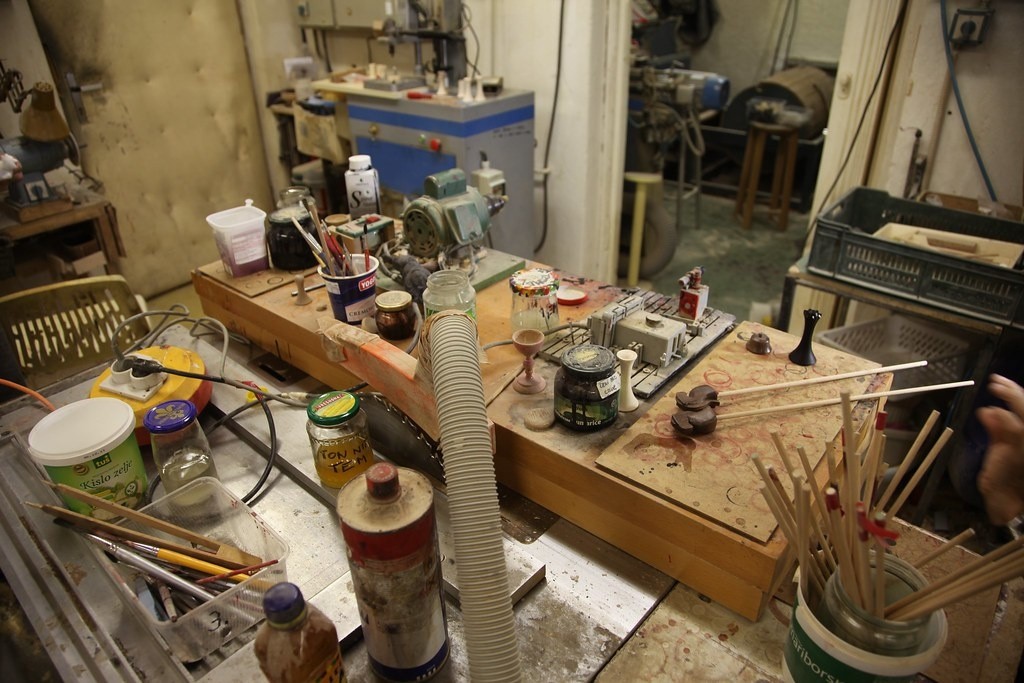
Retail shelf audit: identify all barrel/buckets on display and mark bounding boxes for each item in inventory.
[26,396,149,523]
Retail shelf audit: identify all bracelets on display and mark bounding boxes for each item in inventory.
[992,522,1024,545]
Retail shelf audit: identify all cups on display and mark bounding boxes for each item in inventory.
[318,253,382,325]
[780,545,947,683]
[509,266,559,341]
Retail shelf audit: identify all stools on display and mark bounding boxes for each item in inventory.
[734,119,798,232]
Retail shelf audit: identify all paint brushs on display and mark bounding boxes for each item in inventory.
[289,196,359,277]
[51,518,256,626]
[363,224,369,271]
[117,538,274,590]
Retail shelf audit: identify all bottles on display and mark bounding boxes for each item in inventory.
[818,557,933,657]
[305,391,374,491]
[422,270,477,325]
[252,581,348,683]
[344,154,382,220]
[142,400,220,507]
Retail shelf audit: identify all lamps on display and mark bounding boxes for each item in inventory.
[0,59,70,141]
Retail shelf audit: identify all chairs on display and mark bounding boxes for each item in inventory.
[0,274,153,389]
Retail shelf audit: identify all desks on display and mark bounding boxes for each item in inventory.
[0,186,123,282]
[778,248,1005,526]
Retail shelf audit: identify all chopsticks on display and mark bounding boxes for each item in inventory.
[748,386,1024,629]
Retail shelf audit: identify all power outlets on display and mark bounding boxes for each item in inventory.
[948,7,992,46]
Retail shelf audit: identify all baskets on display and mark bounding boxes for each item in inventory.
[808,187,1024,321]
[816,314,972,400]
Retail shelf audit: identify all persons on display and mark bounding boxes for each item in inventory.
[978,374,1024,548]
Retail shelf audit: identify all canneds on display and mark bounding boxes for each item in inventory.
[307,390,374,489]
[553,344,620,431]
[374,291,415,340]
[265,207,323,270]
[141,400,220,508]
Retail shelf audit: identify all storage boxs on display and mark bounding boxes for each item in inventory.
[89,477,291,664]
[815,184,1024,321]
[817,312,978,401]
[204,203,269,275]
